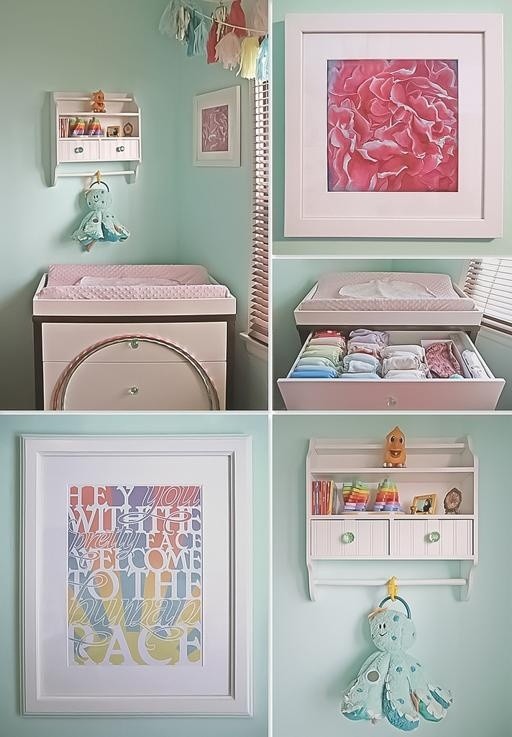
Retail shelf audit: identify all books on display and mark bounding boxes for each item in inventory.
[313,479,338,514]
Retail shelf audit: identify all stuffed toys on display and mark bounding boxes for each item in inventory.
[72,188,130,250]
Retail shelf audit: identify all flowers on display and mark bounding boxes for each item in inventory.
[328,60,460,192]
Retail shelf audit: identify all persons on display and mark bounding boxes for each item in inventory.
[340,609,452,731]
[424,499,431,510]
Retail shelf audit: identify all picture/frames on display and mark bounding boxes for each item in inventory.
[284,9,506,240]
[408,490,438,516]
[17,433,255,718]
[191,84,243,169]
[106,125,120,137]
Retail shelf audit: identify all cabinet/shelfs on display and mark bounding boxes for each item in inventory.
[300,429,483,608]
[30,264,237,408]
[41,88,142,188]
[275,266,509,410]
